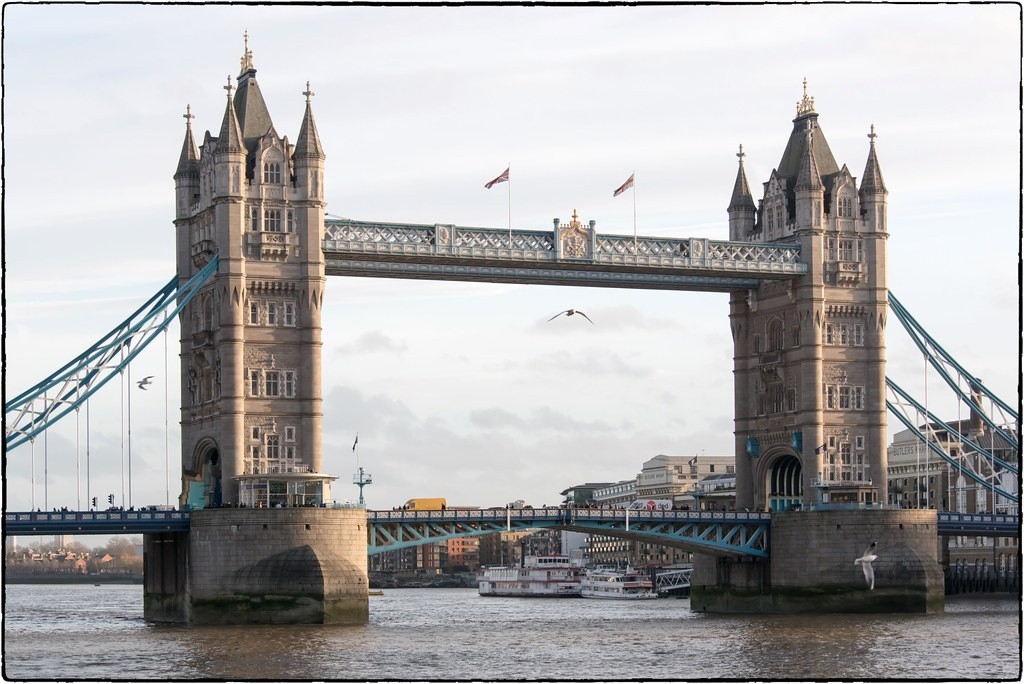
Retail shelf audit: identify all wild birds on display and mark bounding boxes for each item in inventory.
[853,541,879,590]
[136,375,155,391]
[546,308,596,326]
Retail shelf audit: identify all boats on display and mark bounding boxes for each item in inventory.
[580,564,658,599]
[476,554,584,597]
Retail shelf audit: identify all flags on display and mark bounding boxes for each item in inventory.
[689,457,697,466]
[352,436,358,451]
[613,175,634,197]
[485,168,509,189]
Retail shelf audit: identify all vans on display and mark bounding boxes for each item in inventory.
[403,498,447,511]
[625,499,672,511]
